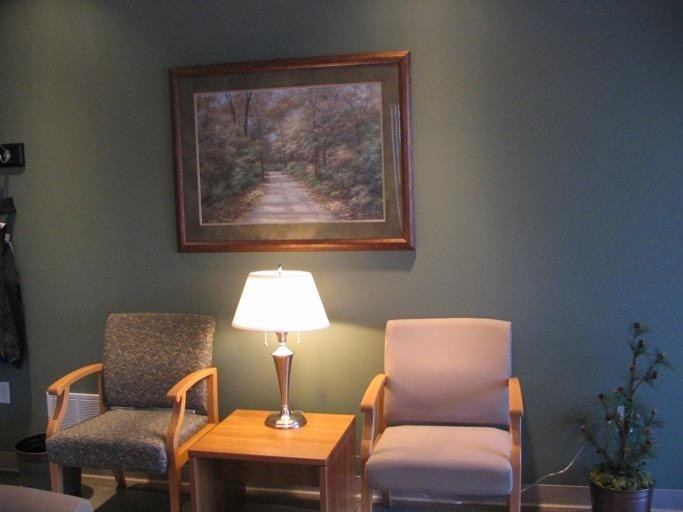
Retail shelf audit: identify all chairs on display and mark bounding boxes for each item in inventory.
[359,317,526,512]
[44,313,220,512]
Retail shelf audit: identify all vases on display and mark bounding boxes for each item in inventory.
[589,477,654,512]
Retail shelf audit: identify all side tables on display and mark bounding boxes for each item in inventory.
[186,408,359,512]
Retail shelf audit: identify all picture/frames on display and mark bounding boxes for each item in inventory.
[167,52,416,255]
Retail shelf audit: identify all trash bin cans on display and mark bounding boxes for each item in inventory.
[14,434,81,499]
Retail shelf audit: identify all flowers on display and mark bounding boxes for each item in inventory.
[576,321,677,491]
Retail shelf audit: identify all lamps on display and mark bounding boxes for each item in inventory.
[231,263,331,430]
